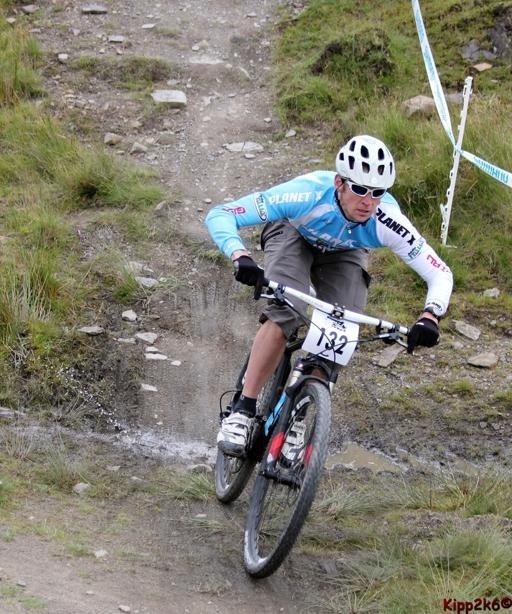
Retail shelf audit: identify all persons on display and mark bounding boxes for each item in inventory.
[204,134,458,467]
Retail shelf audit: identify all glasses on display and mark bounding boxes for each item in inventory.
[344,180,387,199]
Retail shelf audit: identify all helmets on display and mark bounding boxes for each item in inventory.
[335,135,396,189]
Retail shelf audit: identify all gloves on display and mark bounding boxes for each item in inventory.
[233,255,269,300]
[407,317,439,354]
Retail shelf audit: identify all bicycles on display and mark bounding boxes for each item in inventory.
[214,263,440,580]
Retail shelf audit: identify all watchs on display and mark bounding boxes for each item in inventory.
[423,305,444,320]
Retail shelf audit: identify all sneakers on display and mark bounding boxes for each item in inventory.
[216,410,256,458]
[278,419,307,469]
